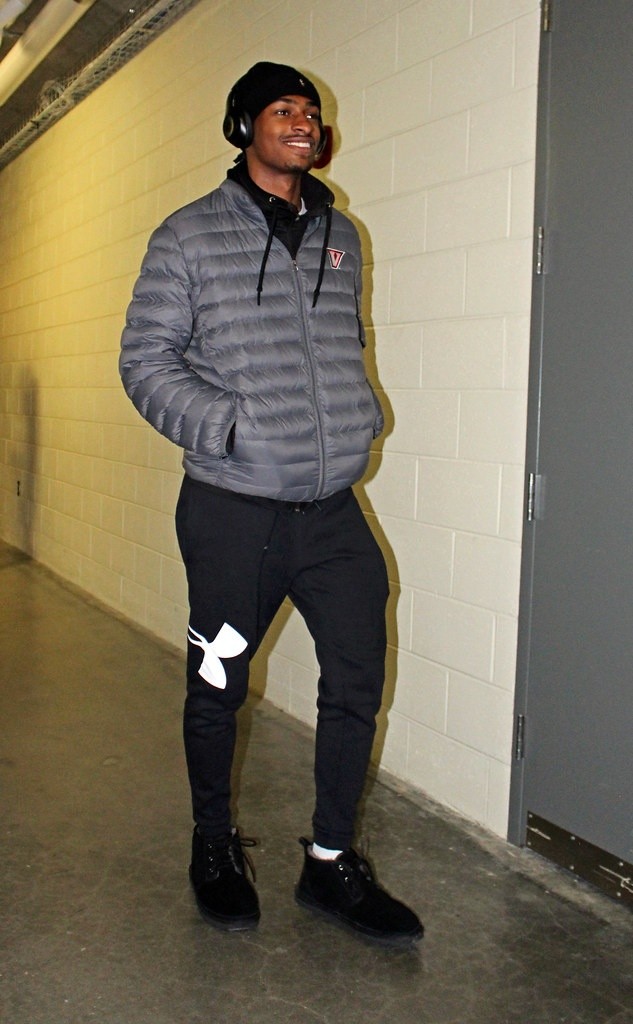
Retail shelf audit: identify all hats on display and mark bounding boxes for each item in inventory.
[228,62,323,127]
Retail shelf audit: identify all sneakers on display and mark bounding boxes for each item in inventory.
[188,824,260,931]
[292,836,425,941]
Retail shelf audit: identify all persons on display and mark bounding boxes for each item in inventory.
[118,59,431,950]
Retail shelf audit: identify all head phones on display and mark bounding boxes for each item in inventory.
[223,67,328,160]
